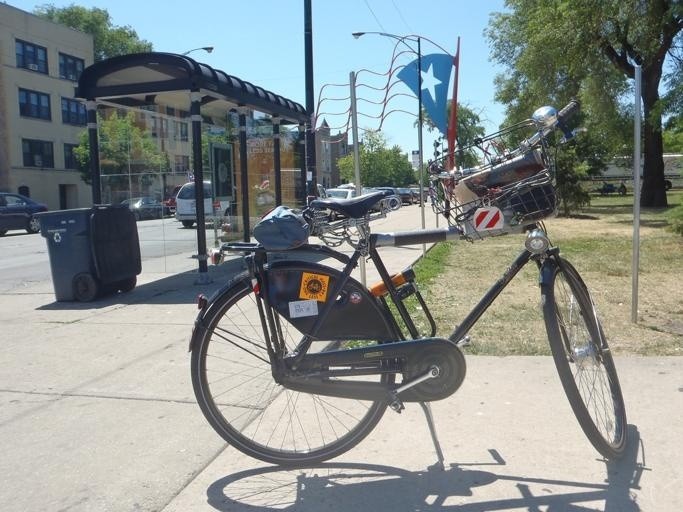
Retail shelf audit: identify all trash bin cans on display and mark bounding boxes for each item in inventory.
[33,203,141,302]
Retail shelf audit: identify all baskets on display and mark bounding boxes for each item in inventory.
[425,117,557,244]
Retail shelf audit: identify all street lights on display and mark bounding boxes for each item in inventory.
[182,46,214,58]
[352,31,427,258]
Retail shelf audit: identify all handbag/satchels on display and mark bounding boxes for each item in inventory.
[254,205,314,251]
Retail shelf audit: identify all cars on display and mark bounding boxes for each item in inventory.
[175,181,236,229]
[166,184,182,217]
[316,182,428,215]
[120,197,170,221]
[0,192,48,237]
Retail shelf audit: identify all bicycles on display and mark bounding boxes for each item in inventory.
[188,100,629,467]
